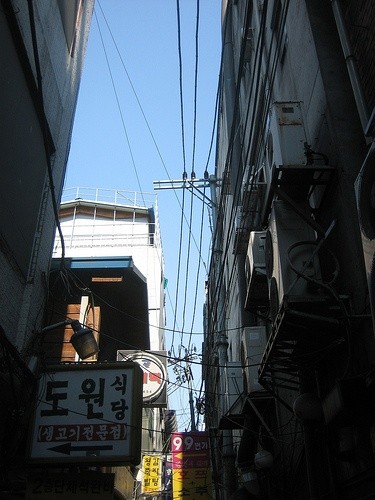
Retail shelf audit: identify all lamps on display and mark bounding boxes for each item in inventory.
[34,317,100,361]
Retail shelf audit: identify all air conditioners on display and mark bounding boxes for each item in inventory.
[221,360,244,417]
[264,197,330,325]
[238,326,274,396]
[261,100,313,196]
[243,227,269,316]
